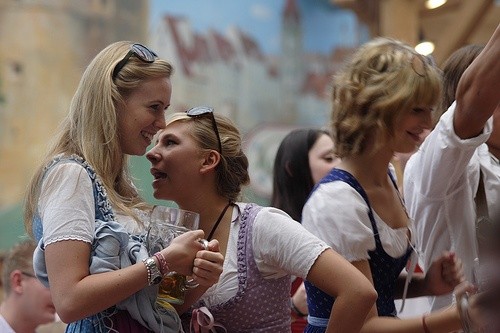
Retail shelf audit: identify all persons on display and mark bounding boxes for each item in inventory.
[270,24,500,333]
[145,105,377,333]
[21,41,225,333]
[0,238,56,333]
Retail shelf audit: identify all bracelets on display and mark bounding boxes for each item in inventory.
[142,251,170,287]
[423,315,430,333]
[290,299,308,317]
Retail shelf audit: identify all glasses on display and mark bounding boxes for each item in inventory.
[410,51,437,78]
[184,105,223,185]
[112,43,159,81]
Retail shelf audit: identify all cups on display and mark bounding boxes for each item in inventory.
[457,258,488,333]
[146,205,209,305]
